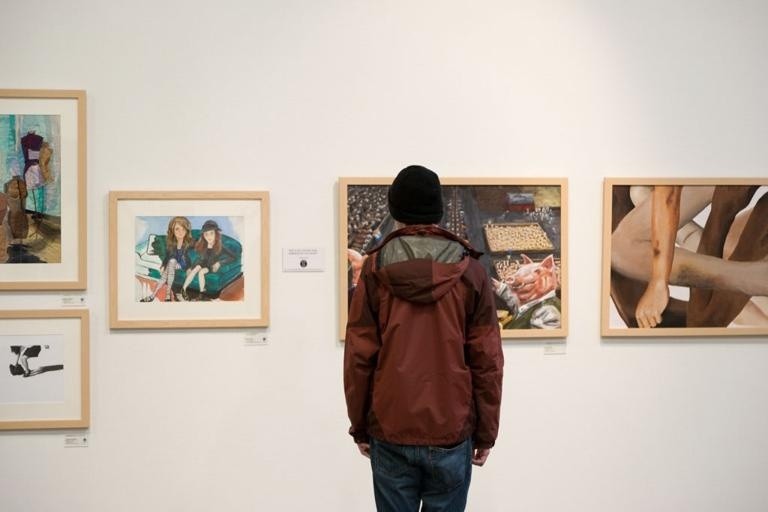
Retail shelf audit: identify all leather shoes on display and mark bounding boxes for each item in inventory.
[140,288,207,302]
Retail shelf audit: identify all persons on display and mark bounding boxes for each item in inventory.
[179,220,239,301]
[139,216,196,302]
[610,184,767,329]
[633,186,683,328]
[342,165,502,512]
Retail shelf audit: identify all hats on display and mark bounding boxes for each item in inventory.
[202,221,218,231]
[388,166,443,225]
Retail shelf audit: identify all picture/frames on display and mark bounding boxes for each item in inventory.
[0,309,93,431]
[338,176,570,343]
[108,190,271,330]
[600,177,768,339]
[0,90,90,291]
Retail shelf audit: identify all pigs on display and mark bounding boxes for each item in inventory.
[504,252,562,305]
